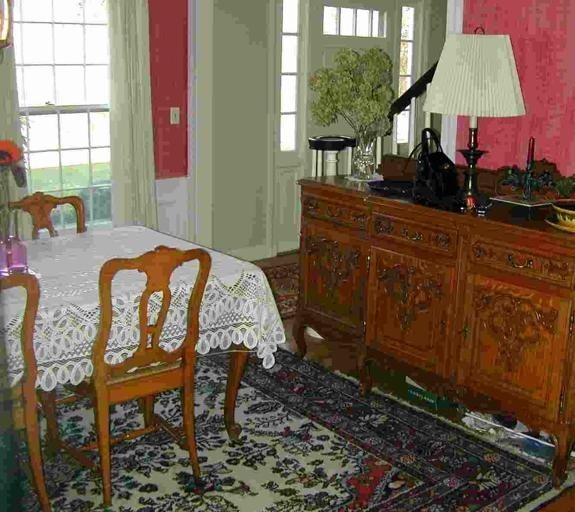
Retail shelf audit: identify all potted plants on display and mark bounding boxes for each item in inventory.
[308,48,396,178]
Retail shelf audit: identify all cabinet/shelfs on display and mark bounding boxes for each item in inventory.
[297,153,575,489]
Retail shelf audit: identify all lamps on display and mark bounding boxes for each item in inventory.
[421,34,527,215]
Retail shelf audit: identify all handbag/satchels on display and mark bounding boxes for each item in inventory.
[409,127,466,214]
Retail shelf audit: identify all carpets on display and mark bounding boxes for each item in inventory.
[262,263,300,319]
[0,342,575,511]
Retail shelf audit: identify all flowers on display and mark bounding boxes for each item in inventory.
[0,138,29,275]
